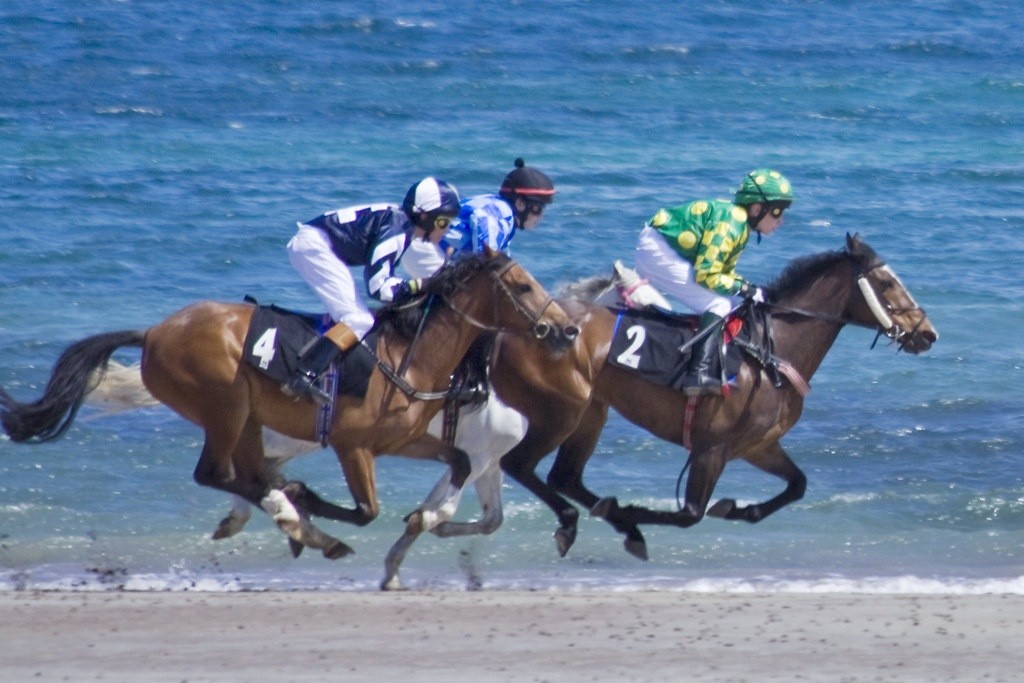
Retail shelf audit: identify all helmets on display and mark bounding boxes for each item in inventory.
[735,168,794,204]
[400,177,461,214]
[499,157,556,204]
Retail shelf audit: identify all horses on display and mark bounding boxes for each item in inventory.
[0,230,940,597]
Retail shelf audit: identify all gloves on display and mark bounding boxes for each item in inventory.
[746,283,771,307]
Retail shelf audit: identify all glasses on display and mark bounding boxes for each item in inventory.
[521,197,543,215]
[434,215,453,232]
[769,207,783,218]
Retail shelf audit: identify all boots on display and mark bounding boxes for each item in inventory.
[281,336,343,403]
[457,340,487,402]
[683,311,742,396]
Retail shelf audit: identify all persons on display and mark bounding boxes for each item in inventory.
[282,176,460,402]
[636,168,793,397]
[400,157,555,278]
